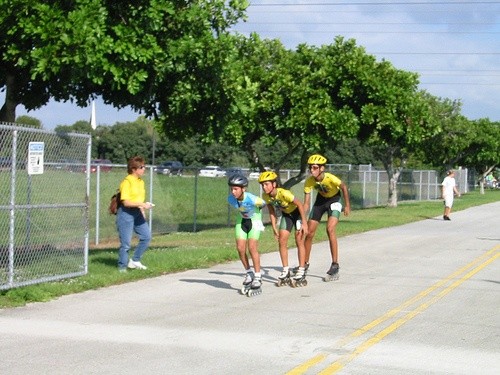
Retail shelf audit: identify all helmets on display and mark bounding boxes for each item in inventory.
[258,171,278,182]
[229,175,248,187]
[307,155,327,165]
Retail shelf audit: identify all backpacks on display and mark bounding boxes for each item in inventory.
[109,191,121,215]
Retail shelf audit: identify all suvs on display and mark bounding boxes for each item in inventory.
[53,159,81,173]
[153,161,183,176]
[82,159,113,173]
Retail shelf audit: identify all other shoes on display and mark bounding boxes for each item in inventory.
[444,215,450,220]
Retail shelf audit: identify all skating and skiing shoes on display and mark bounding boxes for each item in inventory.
[247,276,263,296]
[277,268,290,286]
[241,272,255,294]
[289,262,310,279]
[323,263,340,282]
[289,267,307,288]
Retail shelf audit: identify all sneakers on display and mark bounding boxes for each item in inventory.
[128,258,146,270]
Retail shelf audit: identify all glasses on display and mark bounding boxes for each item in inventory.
[309,167,320,170]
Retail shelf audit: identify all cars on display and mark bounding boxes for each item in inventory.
[249,167,274,178]
[198,166,227,178]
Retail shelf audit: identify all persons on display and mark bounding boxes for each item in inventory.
[258,171,308,280]
[442,169,459,220]
[227,175,266,289]
[292,155,349,276]
[116,157,151,273]
